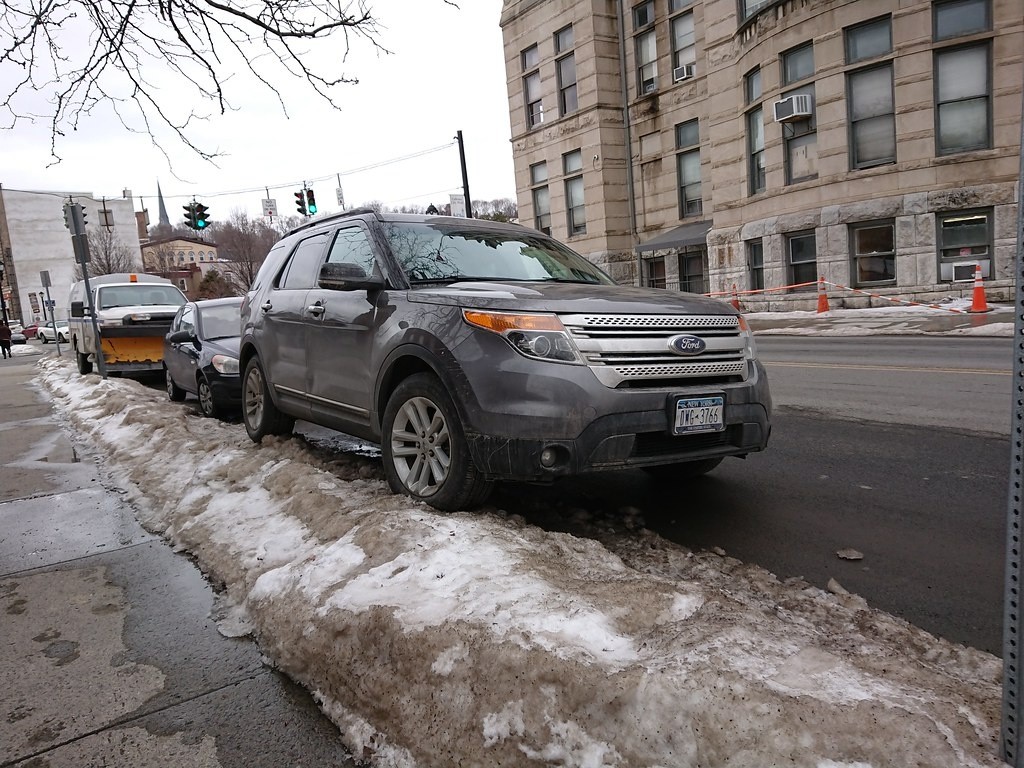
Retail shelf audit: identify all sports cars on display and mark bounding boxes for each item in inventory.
[36,320,70,344]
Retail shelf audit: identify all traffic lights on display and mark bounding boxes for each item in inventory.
[182,203,196,229]
[294,191,307,214]
[193,202,205,229]
[72,203,88,225]
[306,188,317,213]
[202,206,211,227]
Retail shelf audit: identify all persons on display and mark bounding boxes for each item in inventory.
[0,320,12,360]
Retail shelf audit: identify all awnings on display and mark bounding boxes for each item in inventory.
[633,219,714,253]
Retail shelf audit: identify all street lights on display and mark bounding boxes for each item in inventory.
[0,261,9,326]
[38,291,47,320]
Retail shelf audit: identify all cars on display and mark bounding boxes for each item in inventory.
[2,319,27,345]
[21,319,50,341]
[162,296,245,418]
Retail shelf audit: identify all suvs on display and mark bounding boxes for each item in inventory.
[238,204,775,513]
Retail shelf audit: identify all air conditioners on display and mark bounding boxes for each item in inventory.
[773,95,812,123]
[953,262,979,282]
[674,65,694,81]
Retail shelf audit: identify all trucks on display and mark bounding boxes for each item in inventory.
[68,273,190,379]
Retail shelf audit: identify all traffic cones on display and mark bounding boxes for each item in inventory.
[731,284,740,313]
[965,265,995,313]
[816,275,830,313]
